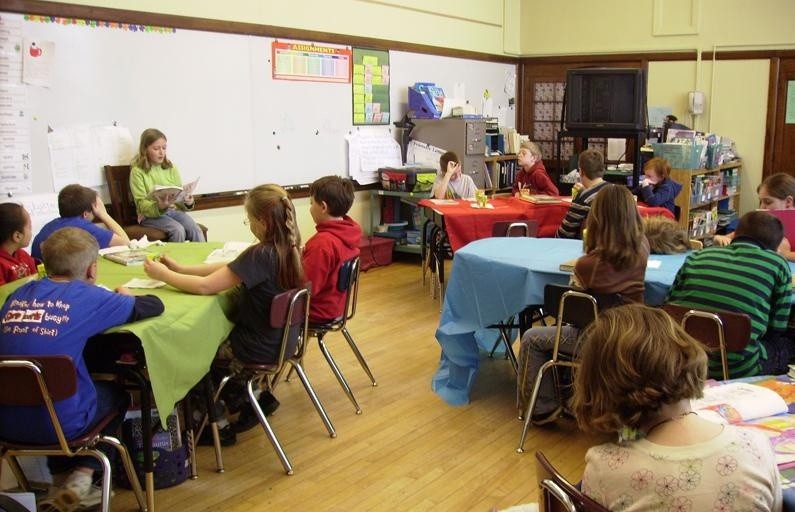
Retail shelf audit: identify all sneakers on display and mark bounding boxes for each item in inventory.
[531,405,562,426]
[230,390,280,433]
[39,485,80,512]
[181,423,235,447]
[74,504,84,512]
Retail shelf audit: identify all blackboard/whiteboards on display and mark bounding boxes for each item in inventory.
[0,0,519,204]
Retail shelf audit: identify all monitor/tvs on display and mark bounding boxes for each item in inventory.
[565,69,643,132]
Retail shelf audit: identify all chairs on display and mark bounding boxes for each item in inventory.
[188,280,337,475]
[491,193,513,200]
[688,237,705,250]
[516,284,624,453]
[0,355,148,512]
[674,205,682,222]
[534,450,613,512]
[104,166,208,243]
[579,218,587,241]
[660,304,751,381]
[266,255,378,415]
[490,220,539,237]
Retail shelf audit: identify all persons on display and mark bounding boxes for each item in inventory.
[0,227,165,512]
[515,184,650,428]
[129,129,205,243]
[428,151,479,201]
[569,303,783,512]
[301,175,362,325]
[511,140,559,198]
[0,202,37,287]
[145,184,308,447]
[555,149,612,238]
[716,172,794,262]
[582,201,691,254]
[631,156,683,212]
[658,210,795,381]
[31,185,130,260]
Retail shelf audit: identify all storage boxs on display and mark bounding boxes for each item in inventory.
[378,168,437,191]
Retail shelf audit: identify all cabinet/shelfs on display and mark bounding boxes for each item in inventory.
[484,155,518,199]
[409,119,486,189]
[365,189,432,255]
[669,159,744,249]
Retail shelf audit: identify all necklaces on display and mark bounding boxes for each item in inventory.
[646,412,698,437]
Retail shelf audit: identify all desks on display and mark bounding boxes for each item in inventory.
[417,195,675,313]
[431,237,795,405]
[0,242,246,512]
[618,375,795,512]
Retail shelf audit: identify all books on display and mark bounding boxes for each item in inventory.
[483,163,493,189]
[523,195,557,200]
[145,177,198,203]
[103,250,150,266]
[497,160,516,188]
[203,241,252,264]
[511,128,531,153]
[559,256,576,271]
[430,199,459,206]
[501,127,511,154]
[427,86,445,112]
[521,194,562,204]
[685,380,794,471]
[788,365,795,380]
